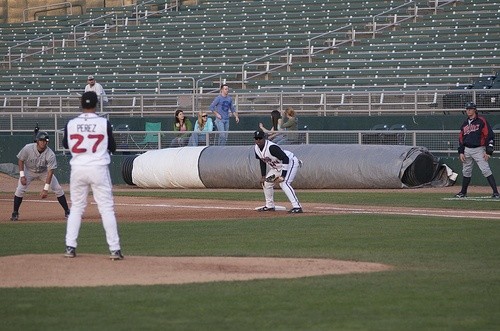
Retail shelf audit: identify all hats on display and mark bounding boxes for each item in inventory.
[87,75,95,80]
[253,130,266,138]
[81,90,98,109]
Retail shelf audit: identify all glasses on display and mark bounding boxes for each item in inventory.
[202,114,208,118]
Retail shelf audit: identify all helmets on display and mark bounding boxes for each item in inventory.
[465,101,477,109]
[36,131,50,141]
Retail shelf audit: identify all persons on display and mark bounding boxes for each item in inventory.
[10,131,70,221]
[79,75,108,112]
[253,131,303,213]
[209,84,240,146]
[61,91,123,261]
[259,110,286,143]
[187,110,213,146]
[280,108,298,144]
[172,110,193,147]
[453,102,500,199]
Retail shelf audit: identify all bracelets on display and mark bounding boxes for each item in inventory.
[20,171,24,178]
[44,184,50,190]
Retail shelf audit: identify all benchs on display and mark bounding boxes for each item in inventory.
[0,0,500,110]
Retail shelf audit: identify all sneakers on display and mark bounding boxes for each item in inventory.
[10,211,19,221]
[110,250,124,260]
[455,192,468,198]
[492,193,500,198]
[63,246,77,257]
[63,210,70,218]
[257,206,275,212]
[288,207,304,213]
